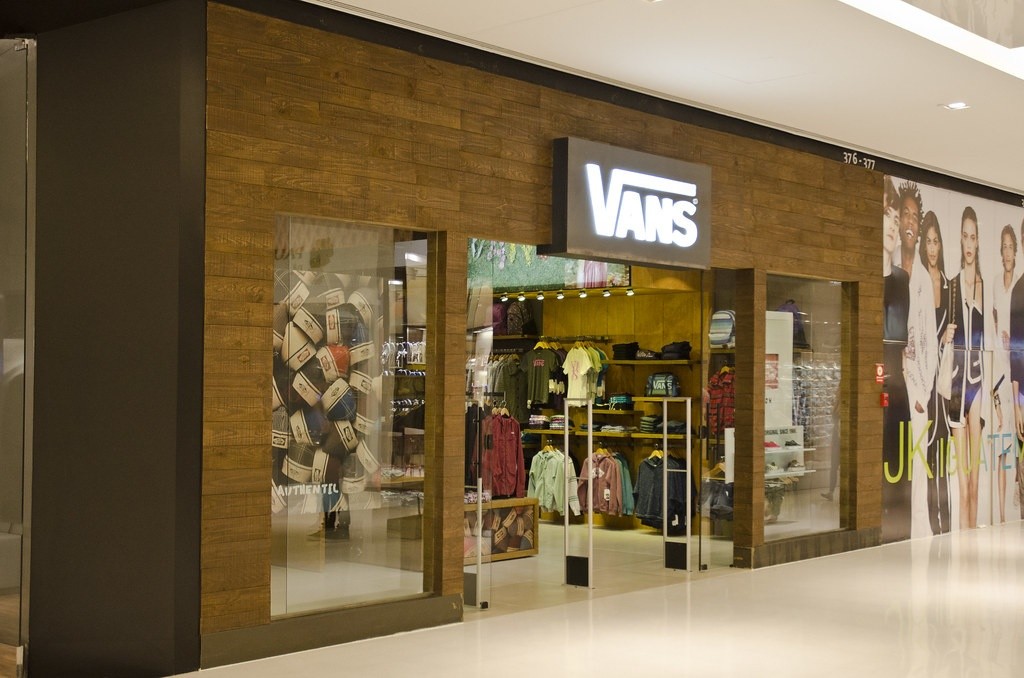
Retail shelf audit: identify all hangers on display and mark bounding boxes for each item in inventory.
[389,398,426,416]
[540,439,565,453]
[572,334,598,350]
[464,347,522,364]
[718,359,735,376]
[701,454,725,480]
[463,391,511,418]
[594,440,621,458]
[763,476,799,488]
[534,335,563,351]
[647,442,682,460]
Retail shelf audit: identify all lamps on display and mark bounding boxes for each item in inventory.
[578,291,587,298]
[626,289,635,296]
[517,293,525,302]
[536,292,545,301]
[557,291,564,300]
[602,290,611,297]
[500,295,508,302]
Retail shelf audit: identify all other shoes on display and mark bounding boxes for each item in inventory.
[821,493,833,500]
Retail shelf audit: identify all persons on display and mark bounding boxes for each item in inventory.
[881,173,1023,546]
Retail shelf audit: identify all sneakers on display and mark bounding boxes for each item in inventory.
[764,441,782,452]
[787,460,806,472]
[767,462,784,473]
[785,440,802,450]
[306,524,350,544]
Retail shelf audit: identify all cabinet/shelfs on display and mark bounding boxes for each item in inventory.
[522,360,702,445]
[709,347,812,443]
[764,447,817,480]
[522,442,540,498]
